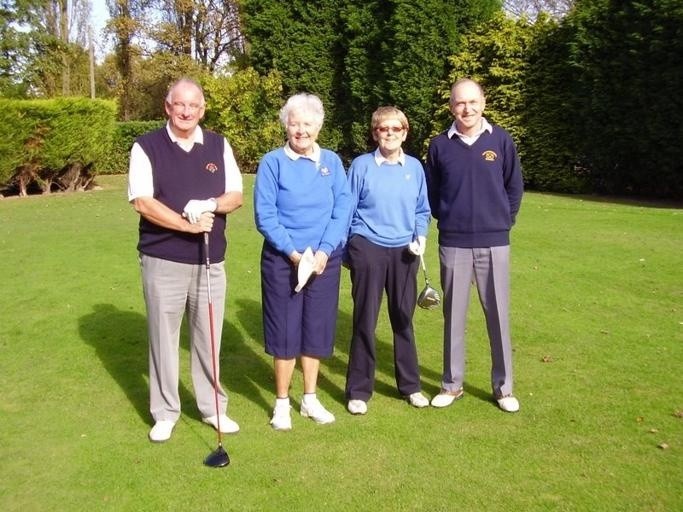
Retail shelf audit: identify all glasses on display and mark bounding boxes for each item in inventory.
[377,125,406,134]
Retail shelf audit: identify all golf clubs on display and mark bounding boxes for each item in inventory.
[201,231,230,467]
[413,228,440,311]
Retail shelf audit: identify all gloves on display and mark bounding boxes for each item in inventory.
[181,198,218,224]
[408,235,426,257]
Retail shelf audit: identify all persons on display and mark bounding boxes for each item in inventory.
[427,77,527,412]
[126,79,241,442]
[252,93,345,431]
[343,106,427,415]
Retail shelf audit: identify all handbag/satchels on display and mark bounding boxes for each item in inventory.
[418,285,441,310]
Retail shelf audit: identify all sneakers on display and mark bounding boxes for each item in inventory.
[347,398,368,414]
[431,388,465,408]
[270,405,292,432]
[201,414,240,434]
[301,402,336,423]
[403,394,430,408]
[494,393,521,414]
[149,417,176,443]
